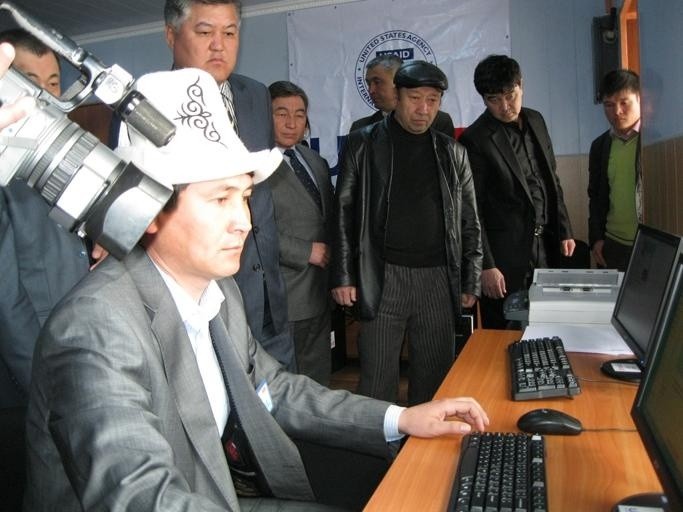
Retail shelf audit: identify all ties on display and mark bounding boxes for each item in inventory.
[283,148,323,216]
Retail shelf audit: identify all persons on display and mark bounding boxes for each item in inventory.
[0,40,35,136]
[23,69,491,510]
[458,56,577,330]
[348,55,456,141]
[107,0,305,457]
[268,80,335,387]
[327,60,485,485]
[587,69,642,273]
[0,27,108,508]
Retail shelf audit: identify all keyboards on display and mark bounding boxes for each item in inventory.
[447,431,546,512]
[509,336,581,400]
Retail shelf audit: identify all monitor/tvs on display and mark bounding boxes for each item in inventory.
[611,254,683,512]
[599,223,682,382]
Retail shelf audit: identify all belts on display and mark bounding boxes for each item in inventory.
[532,224,552,237]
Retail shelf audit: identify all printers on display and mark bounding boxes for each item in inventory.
[505,269,623,322]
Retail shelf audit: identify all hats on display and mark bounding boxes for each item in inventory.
[393,59,449,91]
[114,67,270,188]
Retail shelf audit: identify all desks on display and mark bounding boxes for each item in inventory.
[360,327,667,511]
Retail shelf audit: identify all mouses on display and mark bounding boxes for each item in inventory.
[516,410,582,435]
